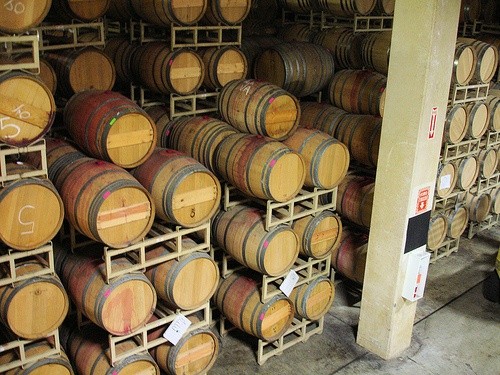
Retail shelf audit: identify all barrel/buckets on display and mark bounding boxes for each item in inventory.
[0,0,500,375]
